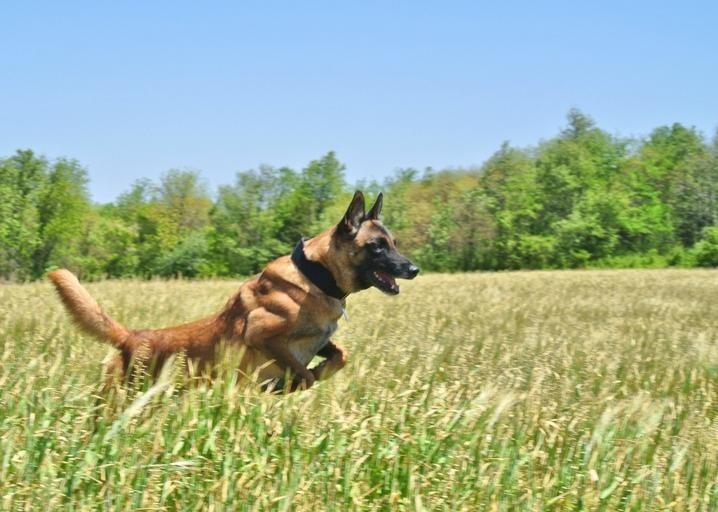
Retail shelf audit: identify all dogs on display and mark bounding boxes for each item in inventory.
[45,189,420,397]
